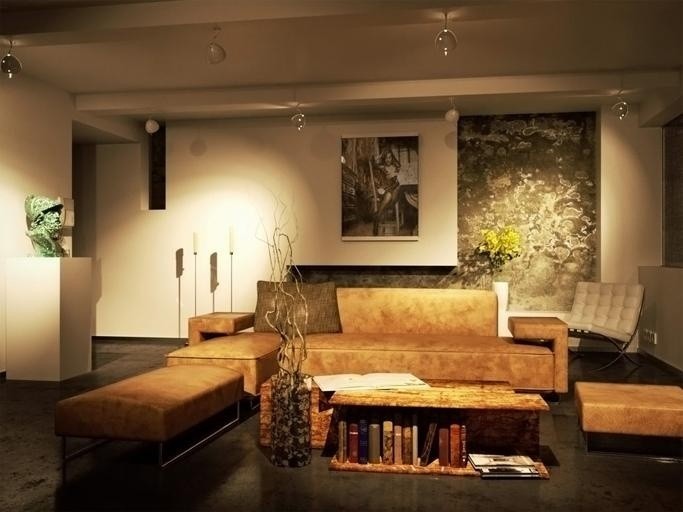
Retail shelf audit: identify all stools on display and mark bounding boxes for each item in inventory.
[569,378,683,457]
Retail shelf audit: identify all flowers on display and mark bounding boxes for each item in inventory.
[476,221,521,275]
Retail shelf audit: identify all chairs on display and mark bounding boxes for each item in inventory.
[569,279,647,371]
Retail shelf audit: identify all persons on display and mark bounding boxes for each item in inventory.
[371,148,418,236]
[24,195,67,257]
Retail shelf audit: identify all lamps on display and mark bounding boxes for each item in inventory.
[444,96,460,124]
[1,38,21,80]
[609,93,632,121]
[144,114,159,136]
[434,10,457,57]
[286,105,306,131]
[205,26,226,64]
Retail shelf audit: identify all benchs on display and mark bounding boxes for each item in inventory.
[53,361,245,474]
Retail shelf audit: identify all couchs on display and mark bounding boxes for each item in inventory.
[164,285,570,401]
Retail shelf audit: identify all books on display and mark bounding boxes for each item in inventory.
[337,418,539,479]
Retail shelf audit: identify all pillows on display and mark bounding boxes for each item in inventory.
[250,279,344,335]
